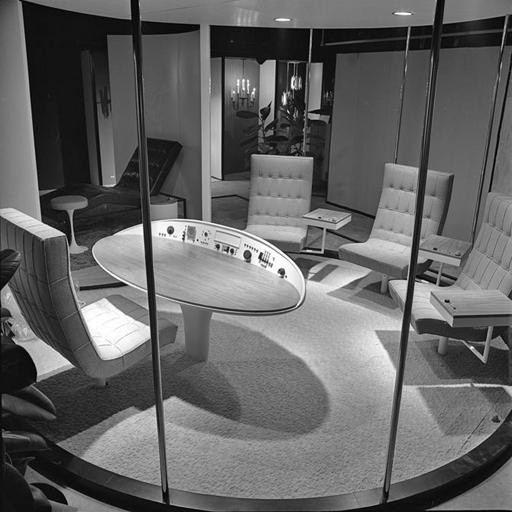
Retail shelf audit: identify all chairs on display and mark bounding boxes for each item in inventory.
[39,136,183,235]
[338,161,456,294]
[243,148,316,254]
[389,192,512,359]
[0,207,178,391]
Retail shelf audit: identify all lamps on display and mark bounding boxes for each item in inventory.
[230,58,257,112]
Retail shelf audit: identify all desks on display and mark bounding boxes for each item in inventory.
[417,233,472,289]
[93,217,307,359]
[427,288,512,365]
[304,208,352,254]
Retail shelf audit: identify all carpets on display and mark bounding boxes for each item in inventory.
[34,187,148,270]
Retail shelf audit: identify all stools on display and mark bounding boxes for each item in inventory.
[50,196,90,256]
